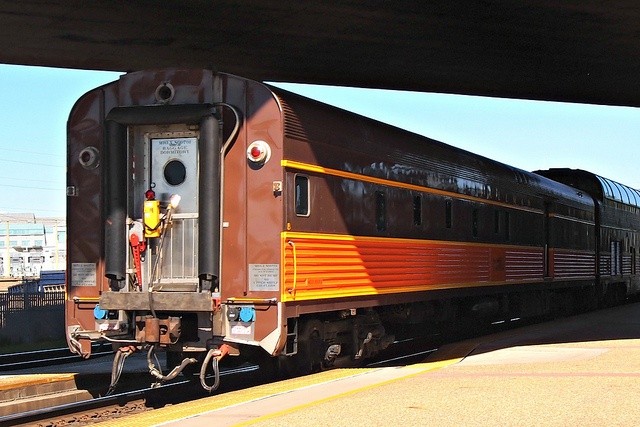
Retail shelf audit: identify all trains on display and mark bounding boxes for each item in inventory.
[65,66,640,395]
